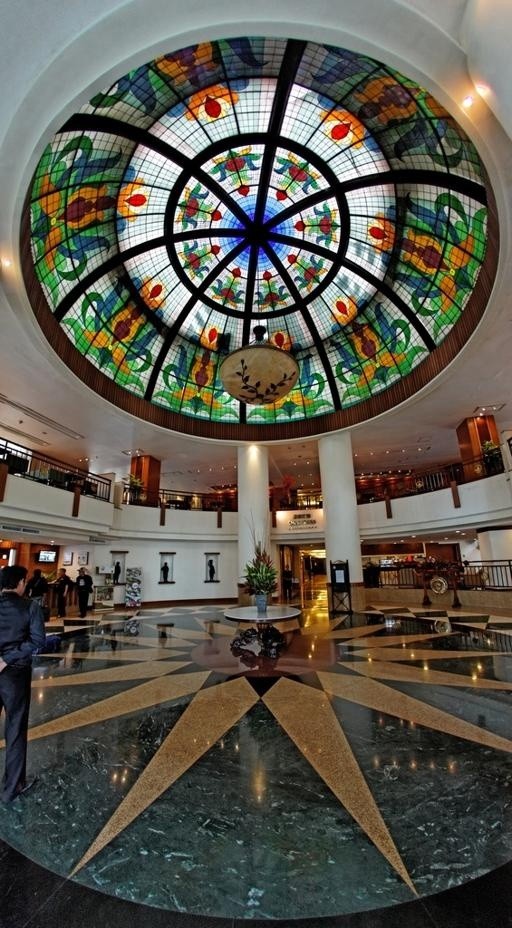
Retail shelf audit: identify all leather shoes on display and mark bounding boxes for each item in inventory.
[19,778,34,794]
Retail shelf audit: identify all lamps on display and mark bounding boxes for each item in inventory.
[220,234,301,406]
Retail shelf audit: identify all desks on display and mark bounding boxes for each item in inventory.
[224,606,302,650]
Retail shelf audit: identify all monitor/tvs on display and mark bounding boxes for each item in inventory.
[38,551,56,563]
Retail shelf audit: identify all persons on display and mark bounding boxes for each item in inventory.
[76,567,93,618]
[113,562,121,583]
[208,559,215,581]
[0,565,46,804]
[282,564,294,604]
[53,568,74,618]
[161,562,169,582]
[26,569,48,608]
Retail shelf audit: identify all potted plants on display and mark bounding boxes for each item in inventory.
[483,441,505,476]
[239,541,280,612]
[127,473,144,505]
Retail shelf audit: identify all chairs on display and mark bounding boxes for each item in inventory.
[0,454,29,478]
[49,468,97,499]
[159,496,233,512]
